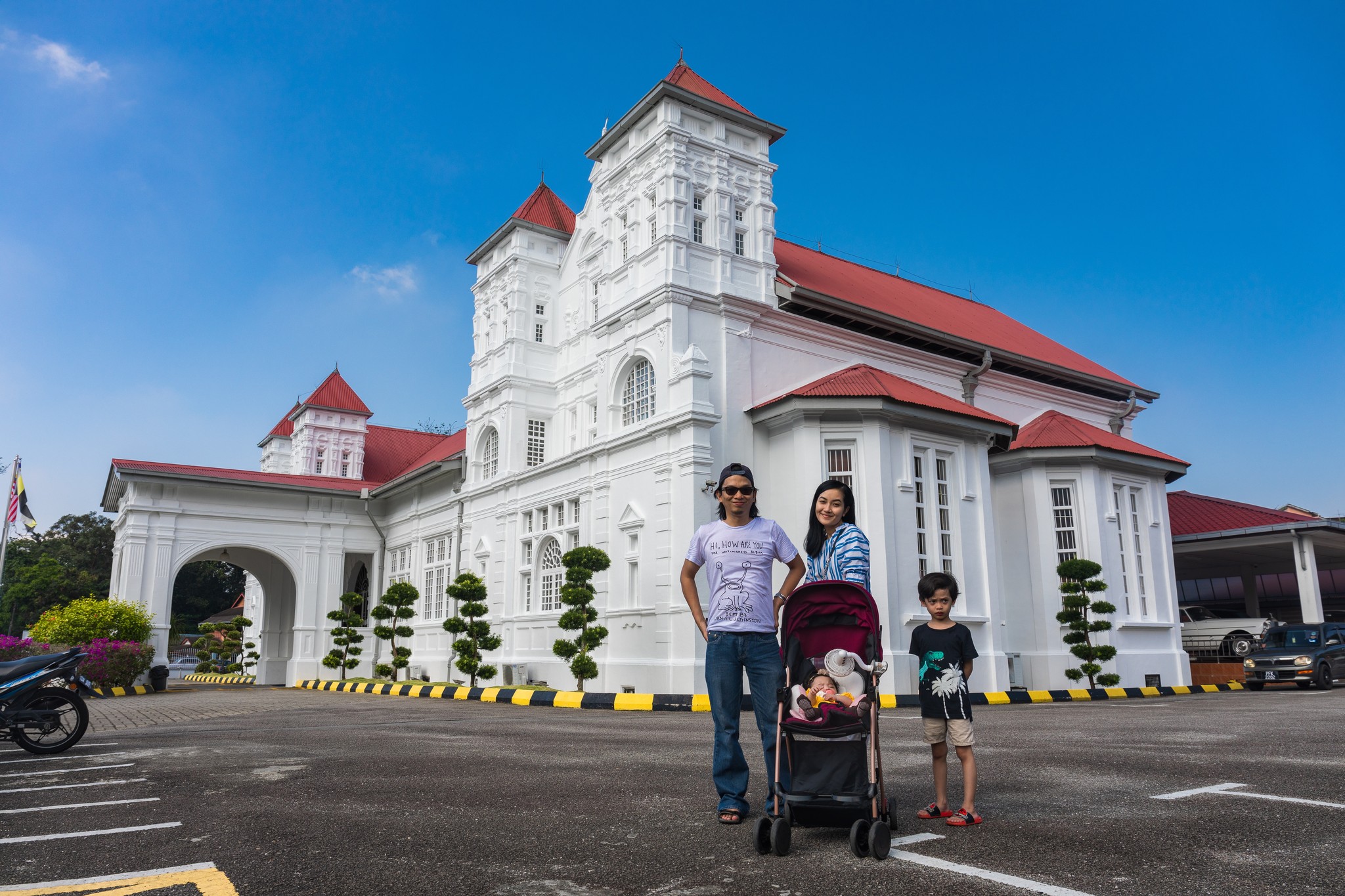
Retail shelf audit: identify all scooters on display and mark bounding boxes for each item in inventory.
[0,646,109,755]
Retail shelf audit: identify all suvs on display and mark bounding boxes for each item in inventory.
[1243,620,1345,691]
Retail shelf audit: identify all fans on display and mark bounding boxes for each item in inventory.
[824,649,888,679]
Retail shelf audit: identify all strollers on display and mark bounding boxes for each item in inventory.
[753,580,897,861]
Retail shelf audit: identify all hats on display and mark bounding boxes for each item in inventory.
[719,463,755,488]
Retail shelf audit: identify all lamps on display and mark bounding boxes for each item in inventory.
[220,547,229,559]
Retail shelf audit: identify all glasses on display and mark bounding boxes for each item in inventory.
[720,486,758,495]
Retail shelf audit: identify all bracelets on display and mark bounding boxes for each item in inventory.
[774,593,787,605]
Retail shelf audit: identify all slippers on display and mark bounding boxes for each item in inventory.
[918,802,953,819]
[946,808,983,826]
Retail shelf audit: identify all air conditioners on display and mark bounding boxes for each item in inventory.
[503,662,528,685]
[406,665,422,680]
[1005,652,1024,687]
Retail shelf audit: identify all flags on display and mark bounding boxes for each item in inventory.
[7,473,18,523]
[14,467,37,533]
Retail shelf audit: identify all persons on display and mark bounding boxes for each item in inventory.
[796,671,872,722]
[803,479,871,595]
[680,463,806,826]
[908,572,982,826]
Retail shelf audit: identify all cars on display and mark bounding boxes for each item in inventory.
[1179,606,1288,661]
[169,658,202,668]
[205,659,235,674]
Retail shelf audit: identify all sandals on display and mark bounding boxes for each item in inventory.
[718,808,743,824]
[768,813,795,821]
